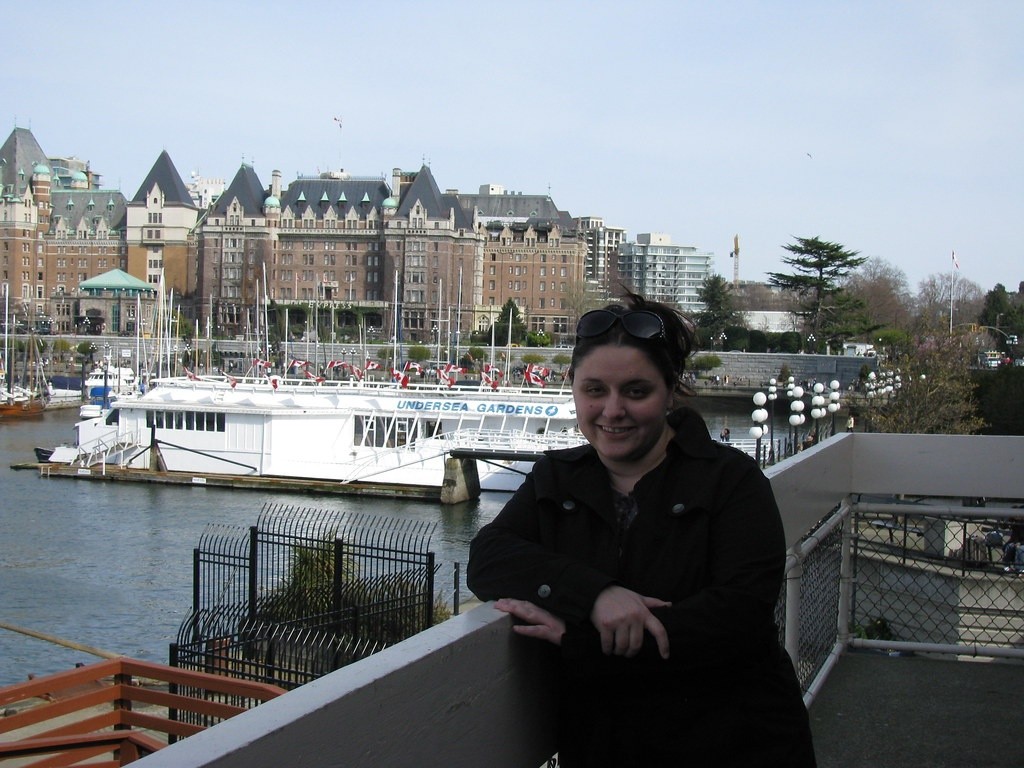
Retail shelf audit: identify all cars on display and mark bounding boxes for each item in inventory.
[969,352,1024,369]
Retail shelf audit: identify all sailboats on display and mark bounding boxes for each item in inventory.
[0,261,594,495]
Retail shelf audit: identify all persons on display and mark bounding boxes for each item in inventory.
[720,429,730,442]
[802,428,817,450]
[419,367,566,382]
[466,300,818,768]
[683,373,729,385]
[845,414,854,432]
[798,378,817,392]
[850,378,866,392]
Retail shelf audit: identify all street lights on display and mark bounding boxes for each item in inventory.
[864,367,902,433]
[140,318,148,336]
[786,375,796,454]
[827,380,841,436]
[710,336,713,351]
[996,313,1004,352]
[787,386,806,455]
[340,347,347,378]
[810,382,827,445]
[807,334,816,354]
[537,328,545,346]
[348,347,358,374]
[747,391,770,470]
[83,315,91,335]
[766,376,779,465]
[718,332,728,352]
[39,311,46,336]
[88,342,98,368]
[368,324,377,343]
[878,337,883,357]
[431,324,439,344]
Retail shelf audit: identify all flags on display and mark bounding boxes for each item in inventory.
[438,364,467,388]
[350,360,381,381]
[185,369,200,381]
[255,359,272,366]
[222,371,236,388]
[524,364,549,388]
[390,361,423,389]
[481,364,503,390]
[304,370,325,382]
[328,361,346,368]
[292,360,315,367]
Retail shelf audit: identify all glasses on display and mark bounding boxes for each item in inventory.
[576,310,679,378]
